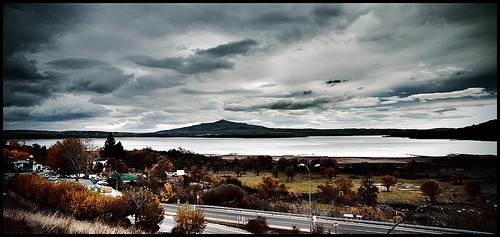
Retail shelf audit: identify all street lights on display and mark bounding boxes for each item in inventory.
[298,163,320,232]
